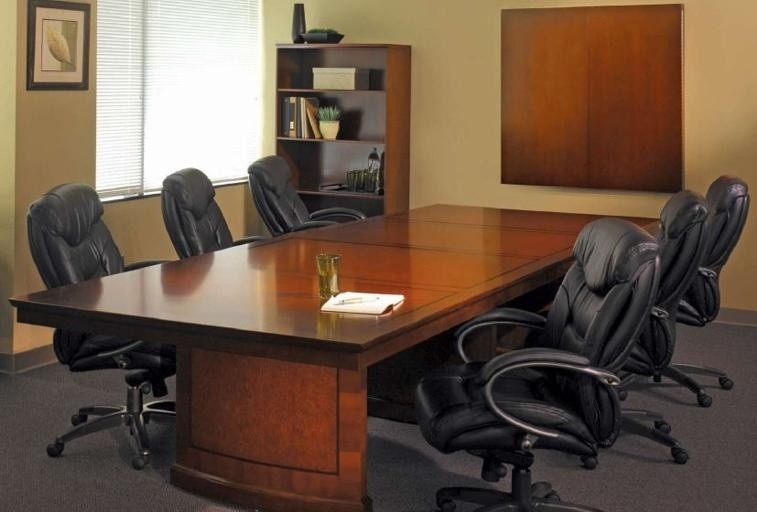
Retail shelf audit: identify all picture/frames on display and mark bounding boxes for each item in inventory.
[24,0,92,95]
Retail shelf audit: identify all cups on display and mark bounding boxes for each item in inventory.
[346,170,367,193]
[316,252,342,301]
[316,301,340,341]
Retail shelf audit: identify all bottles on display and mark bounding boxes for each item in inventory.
[368,151,384,195]
[291,3,306,44]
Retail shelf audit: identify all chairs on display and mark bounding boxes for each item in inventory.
[21,180,181,474]
[157,165,268,259]
[616,172,752,409]
[407,210,672,512]
[245,152,367,235]
[520,187,712,465]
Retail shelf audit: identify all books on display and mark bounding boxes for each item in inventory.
[279,95,322,139]
[320,290,405,314]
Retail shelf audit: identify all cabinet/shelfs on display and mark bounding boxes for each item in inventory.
[272,43,414,224]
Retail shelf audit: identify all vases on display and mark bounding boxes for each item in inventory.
[288,2,310,44]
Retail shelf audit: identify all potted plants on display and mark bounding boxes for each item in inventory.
[316,103,344,141]
[297,27,346,44]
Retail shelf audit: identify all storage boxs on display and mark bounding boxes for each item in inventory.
[310,66,372,91]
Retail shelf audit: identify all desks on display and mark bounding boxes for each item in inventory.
[1,201,671,512]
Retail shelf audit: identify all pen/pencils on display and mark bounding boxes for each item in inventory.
[342,297,379,304]
